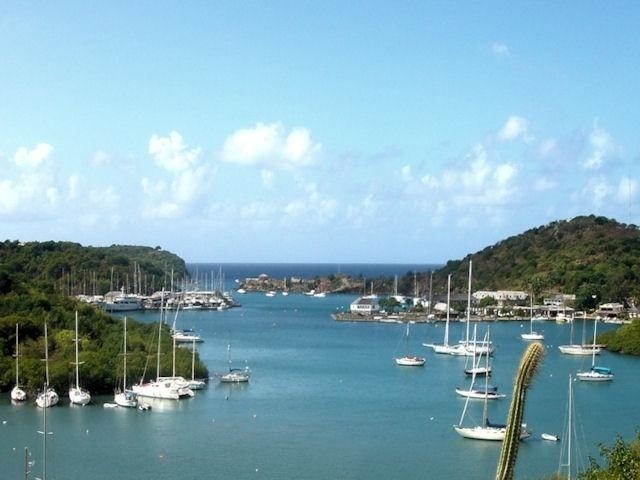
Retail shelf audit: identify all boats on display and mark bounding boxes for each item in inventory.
[591,365,612,374]
[531,313,550,321]
[541,433,560,441]
[455,387,508,399]
[262,287,327,298]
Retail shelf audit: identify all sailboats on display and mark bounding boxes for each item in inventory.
[8,257,251,418]
[453,323,533,441]
[575,319,614,381]
[520,290,545,340]
[422,258,496,356]
[391,321,426,367]
[554,289,572,323]
[557,311,607,356]
[462,323,492,376]
